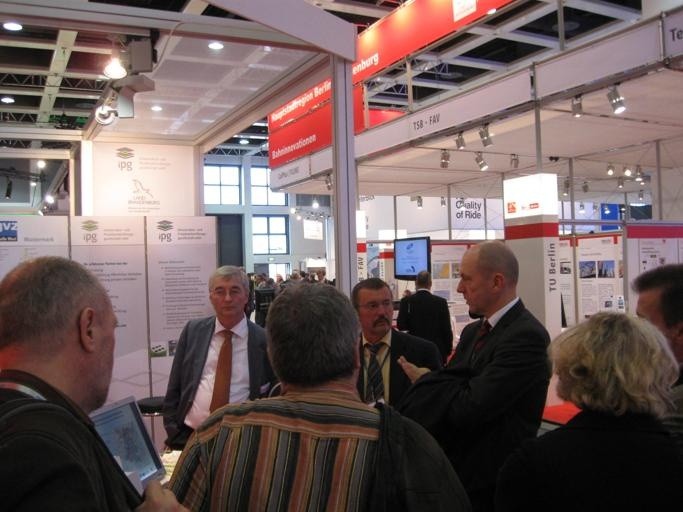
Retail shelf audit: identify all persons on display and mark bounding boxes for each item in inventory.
[392,289,412,312]
[394,270,455,367]
[1,255,189,510]
[164,266,281,450]
[629,261,683,382]
[490,312,681,509]
[165,282,475,510]
[248,267,337,329]
[347,277,446,410]
[397,240,553,512]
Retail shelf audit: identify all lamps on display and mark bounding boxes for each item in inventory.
[570,96,583,118]
[607,82,625,114]
[439,123,495,171]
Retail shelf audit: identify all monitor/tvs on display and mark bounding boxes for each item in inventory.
[394,236,431,281]
[88,396,166,489]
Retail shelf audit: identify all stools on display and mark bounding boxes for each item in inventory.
[138,396,170,443]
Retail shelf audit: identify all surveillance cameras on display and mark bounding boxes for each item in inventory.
[550,156,559,162]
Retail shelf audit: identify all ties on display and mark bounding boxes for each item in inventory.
[367,342,384,404]
[209,330,233,413]
[474,321,491,357]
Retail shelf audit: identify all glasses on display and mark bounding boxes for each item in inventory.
[209,287,247,297]
[357,301,393,312]
